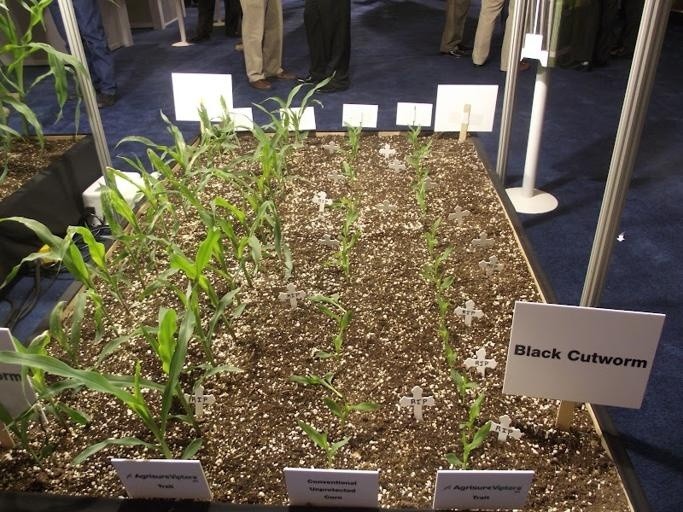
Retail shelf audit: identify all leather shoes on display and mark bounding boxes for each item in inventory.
[226,29,241,37]
[297,73,315,84]
[474,47,494,68]
[448,50,465,57]
[459,43,473,50]
[315,77,348,94]
[251,79,272,89]
[274,70,296,79]
[186,34,211,44]
[519,61,530,71]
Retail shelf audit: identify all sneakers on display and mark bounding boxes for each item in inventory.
[98,88,118,108]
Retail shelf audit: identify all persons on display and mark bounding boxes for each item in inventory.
[186,0,243,43]
[45,0,121,109]
[239,0,295,90]
[438,0,645,72]
[295,0,352,93]
